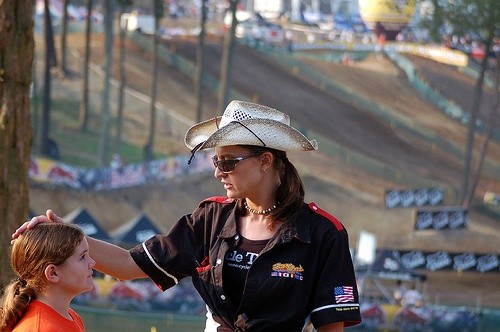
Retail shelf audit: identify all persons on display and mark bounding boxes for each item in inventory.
[11,100,361,332]
[0,222,96,332]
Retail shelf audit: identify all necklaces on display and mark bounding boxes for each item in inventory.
[244,200,282,217]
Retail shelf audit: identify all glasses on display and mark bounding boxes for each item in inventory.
[211,152,263,172]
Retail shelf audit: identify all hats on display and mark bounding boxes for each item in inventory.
[183,100,319,166]
